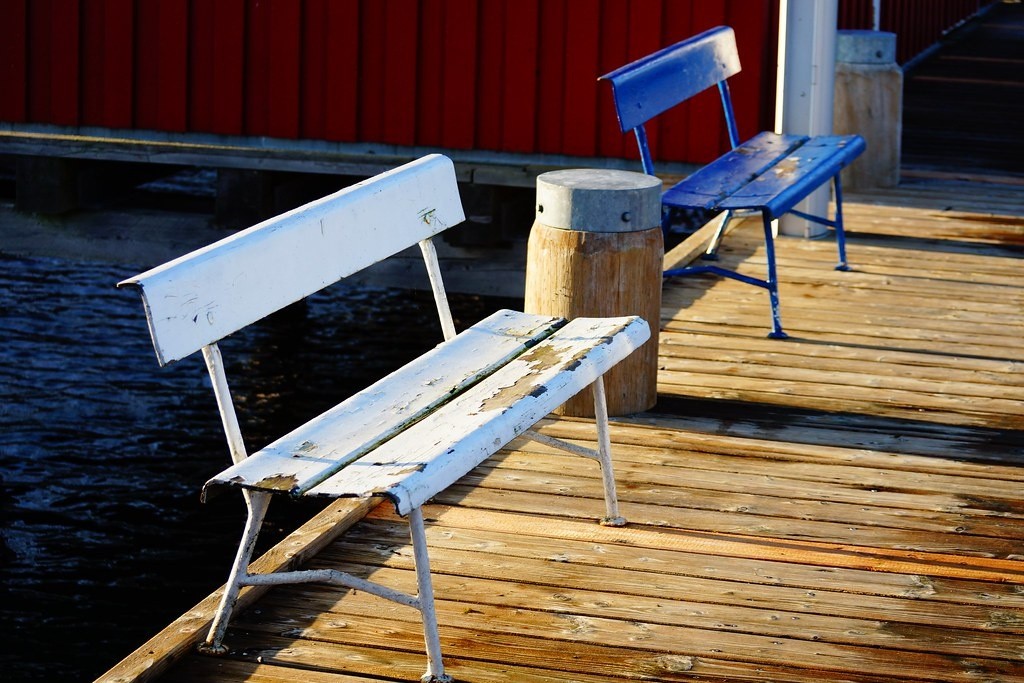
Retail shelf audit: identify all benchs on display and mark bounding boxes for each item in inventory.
[597,26,866,339]
[117,153,650,683]
